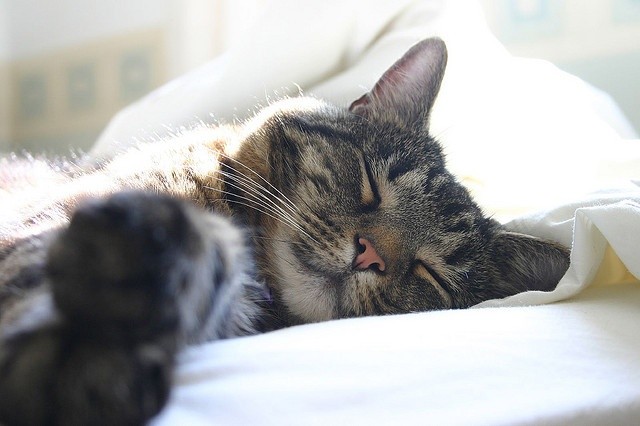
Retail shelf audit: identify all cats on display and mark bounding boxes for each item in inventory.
[0,37,570,426]
